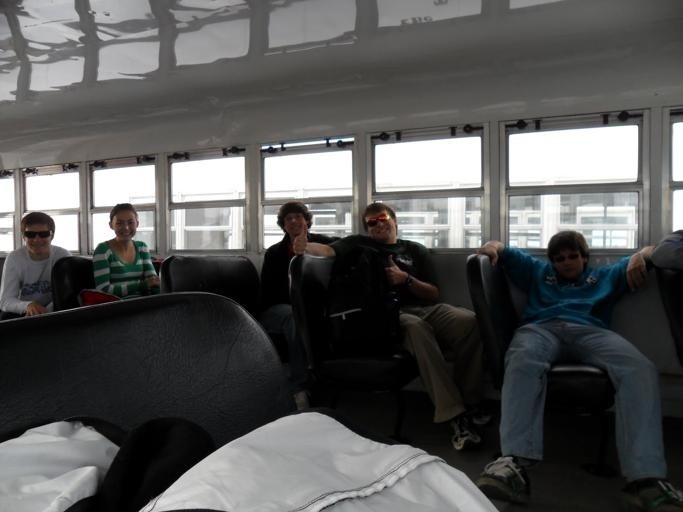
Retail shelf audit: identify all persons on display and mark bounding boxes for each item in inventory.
[254,198,340,413]
[477,230,681,510]
[89,202,161,302]
[0,211,77,319]
[289,203,494,454]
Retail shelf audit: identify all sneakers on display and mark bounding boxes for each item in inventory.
[449,416,491,451]
[621,481,683,512]
[477,456,531,505]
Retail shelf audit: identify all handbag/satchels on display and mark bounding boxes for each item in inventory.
[320,250,400,359]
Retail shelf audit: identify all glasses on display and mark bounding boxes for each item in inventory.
[366,214,389,226]
[24,230,52,238]
[554,253,579,262]
